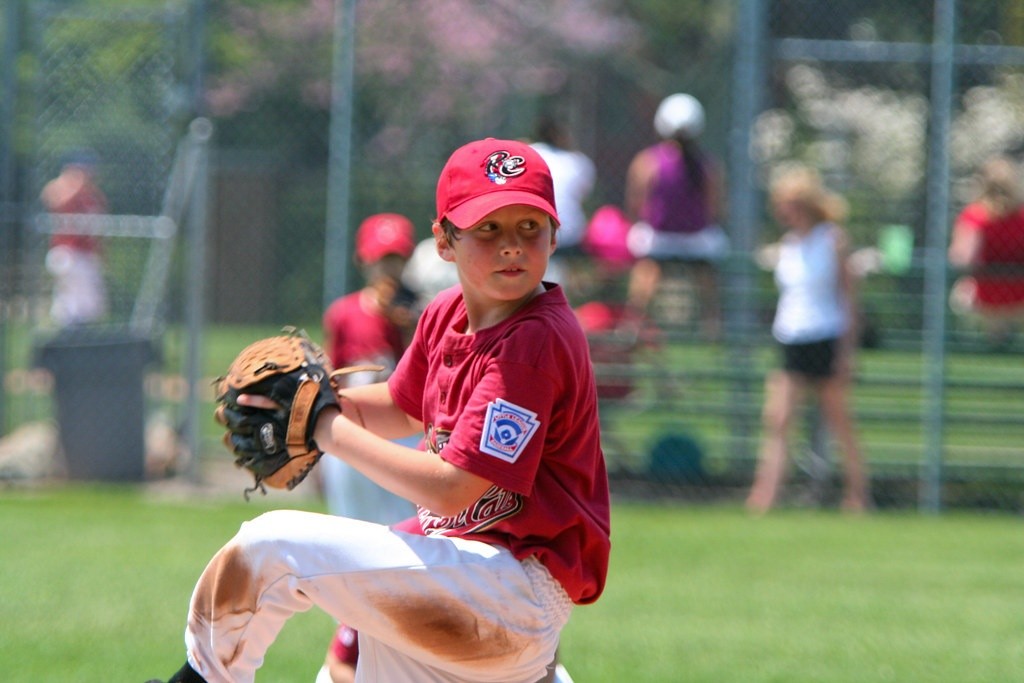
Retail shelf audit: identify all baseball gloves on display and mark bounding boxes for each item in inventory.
[212,329,345,493]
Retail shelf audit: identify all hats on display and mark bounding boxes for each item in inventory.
[355,212,416,265]
[654,93,707,138]
[435,137,562,231]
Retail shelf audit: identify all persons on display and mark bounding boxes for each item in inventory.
[12,142,111,395]
[141,137,612,683]
[745,160,872,516]
[392,111,597,330]
[308,213,576,683]
[950,140,1023,328]
[606,92,733,368]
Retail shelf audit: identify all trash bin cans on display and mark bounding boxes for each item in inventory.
[32,322,159,484]
